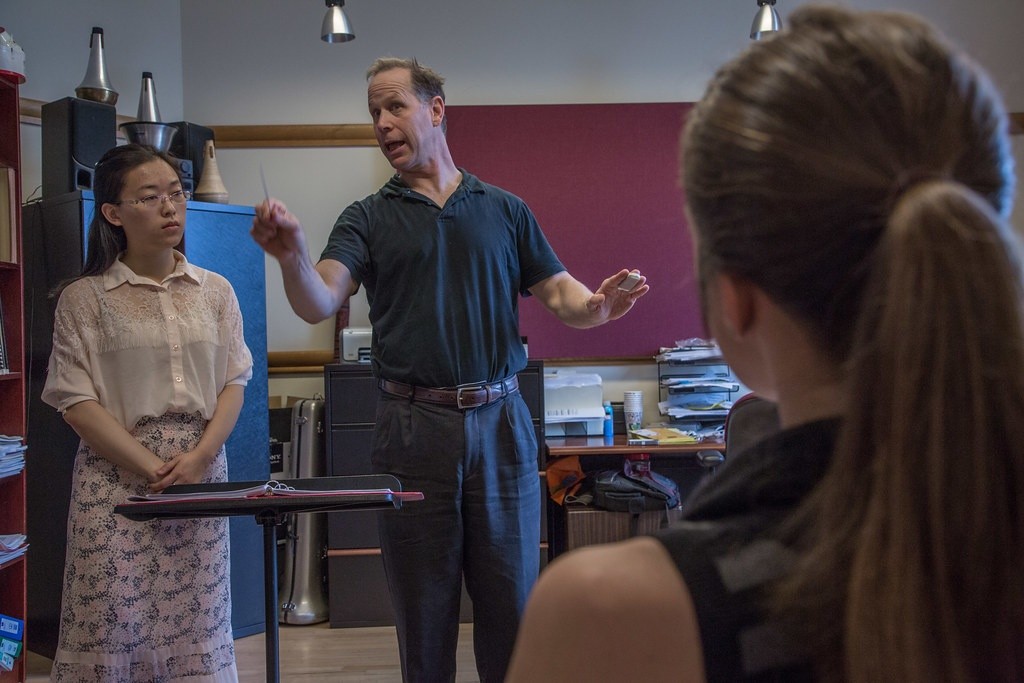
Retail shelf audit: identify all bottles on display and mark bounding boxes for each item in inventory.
[603,400,614,437]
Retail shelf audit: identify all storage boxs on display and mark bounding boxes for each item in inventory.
[564,503,663,551]
[271,394,314,546]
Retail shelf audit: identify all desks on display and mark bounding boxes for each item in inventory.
[543,436,725,562]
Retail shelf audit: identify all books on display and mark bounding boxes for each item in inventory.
[0,534,30,564]
[0,435,27,479]
[123,481,425,502]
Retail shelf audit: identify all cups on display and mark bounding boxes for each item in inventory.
[623,391,644,434]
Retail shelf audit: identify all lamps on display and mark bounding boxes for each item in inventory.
[321,0,355,44]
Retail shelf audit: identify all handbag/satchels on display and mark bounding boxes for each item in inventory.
[593,467,680,512]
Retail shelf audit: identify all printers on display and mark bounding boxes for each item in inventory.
[544,373,605,436]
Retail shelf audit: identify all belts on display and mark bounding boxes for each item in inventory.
[379,372,519,411]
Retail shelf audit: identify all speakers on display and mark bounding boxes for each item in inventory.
[169,121,215,192]
[41,96,117,198]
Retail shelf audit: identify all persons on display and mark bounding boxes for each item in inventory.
[248,56,651,683]
[502,4,1024,683]
[39,143,254,683]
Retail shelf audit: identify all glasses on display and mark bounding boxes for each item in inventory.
[108,190,193,207]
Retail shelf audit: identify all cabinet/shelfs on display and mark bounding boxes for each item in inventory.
[323,360,548,630]
[656,346,739,426]
[0,70,25,683]
